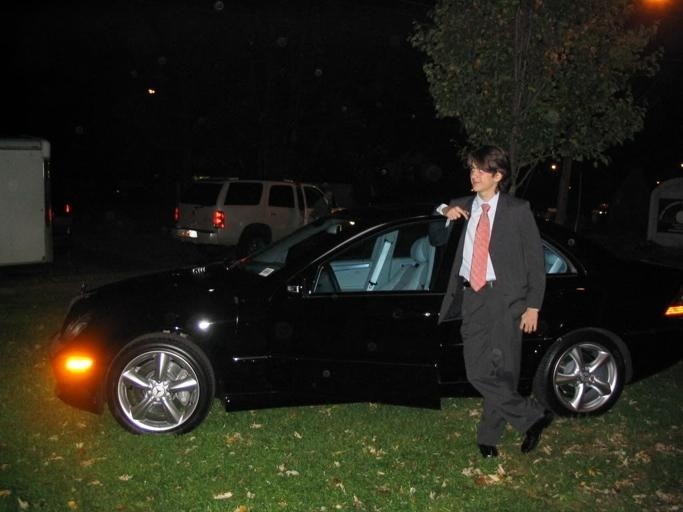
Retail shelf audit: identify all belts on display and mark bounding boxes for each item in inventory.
[462,281,495,287]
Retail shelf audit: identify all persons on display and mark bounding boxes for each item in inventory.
[309,190,347,221]
[429,145,553,458]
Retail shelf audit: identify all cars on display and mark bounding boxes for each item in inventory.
[45,202,683,435]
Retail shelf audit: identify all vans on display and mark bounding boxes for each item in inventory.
[170,177,342,255]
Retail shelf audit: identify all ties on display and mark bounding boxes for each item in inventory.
[470,203,491,293]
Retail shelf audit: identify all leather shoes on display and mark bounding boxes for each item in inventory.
[478,442,497,460]
[520,410,553,454]
